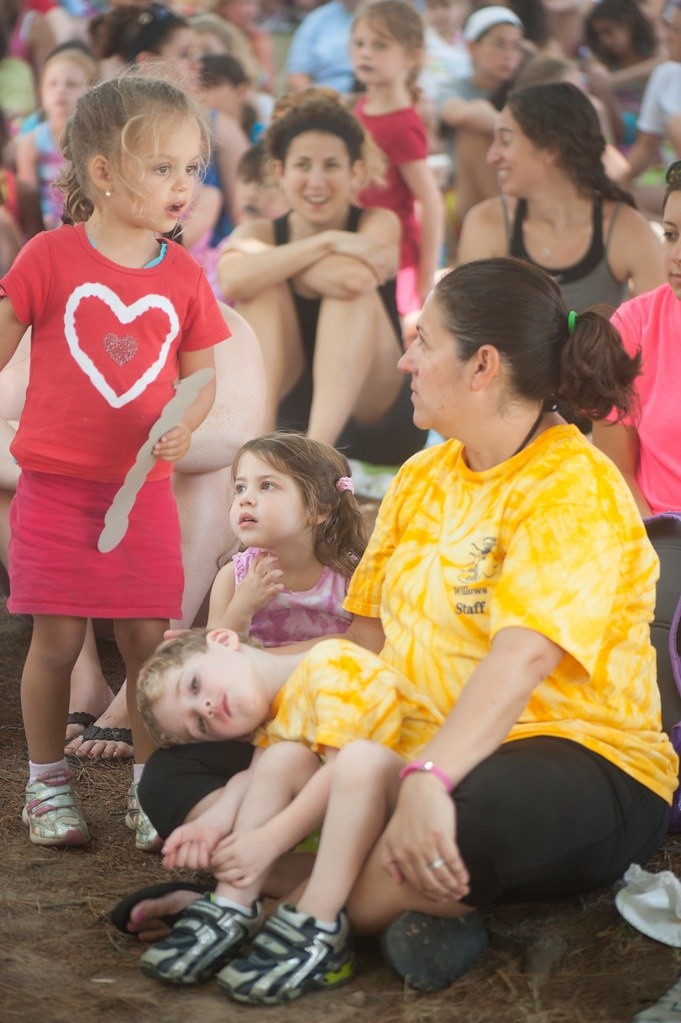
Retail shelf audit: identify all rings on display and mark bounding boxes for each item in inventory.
[429,856,448,875]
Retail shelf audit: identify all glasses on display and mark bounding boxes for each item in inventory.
[666,160,681,186]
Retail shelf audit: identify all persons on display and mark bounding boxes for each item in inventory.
[208,433,369,647]
[0,77,233,851]
[592,162,681,830]
[109,257,681,999]
[136,628,446,1006]
[0,0,680,761]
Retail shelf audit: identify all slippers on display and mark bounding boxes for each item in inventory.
[63,725,134,765]
[65,710,98,746]
[378,909,488,992]
[111,881,218,944]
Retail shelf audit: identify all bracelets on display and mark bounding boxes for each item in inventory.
[397,760,456,796]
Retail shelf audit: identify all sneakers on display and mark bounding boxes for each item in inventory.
[140,891,266,985]
[123,781,166,850]
[215,901,357,1005]
[21,769,90,847]
